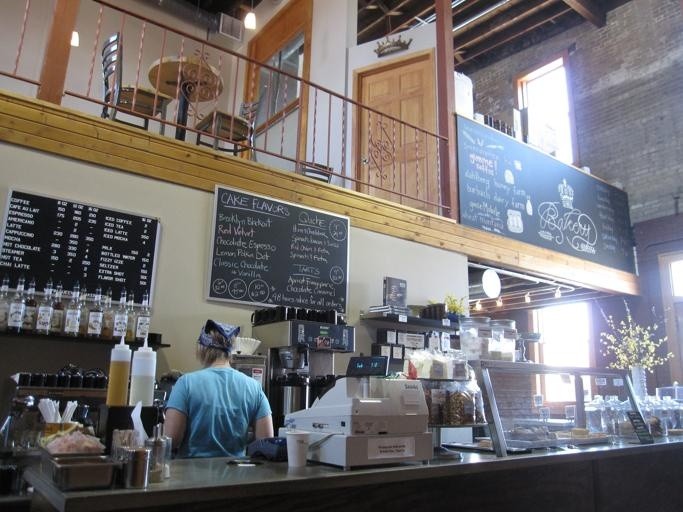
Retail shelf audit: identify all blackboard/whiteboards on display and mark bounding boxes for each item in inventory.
[455,112,640,278]
[0,188,161,309]
[204,182,351,318]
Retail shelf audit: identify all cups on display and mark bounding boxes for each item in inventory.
[286,431,310,467]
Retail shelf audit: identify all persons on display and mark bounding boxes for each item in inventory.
[164,319,274,459]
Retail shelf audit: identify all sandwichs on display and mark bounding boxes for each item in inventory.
[428,358,469,381]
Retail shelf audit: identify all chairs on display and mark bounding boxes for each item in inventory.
[300,162,334,185]
[101,32,172,136]
[196,102,259,162]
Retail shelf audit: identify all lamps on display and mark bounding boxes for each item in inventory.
[482,268,504,298]
[244,0,257,29]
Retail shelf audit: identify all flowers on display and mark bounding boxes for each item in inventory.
[597,297,674,373]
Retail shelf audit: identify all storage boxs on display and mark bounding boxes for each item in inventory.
[39,448,104,478]
[51,456,114,491]
[656,388,683,400]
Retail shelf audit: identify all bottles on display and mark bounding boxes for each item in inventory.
[126,292,136,341]
[0,279,11,330]
[124,449,150,489]
[134,294,151,342]
[144,424,172,482]
[115,447,130,488]
[17,303,18,304]
[50,283,64,335]
[113,291,128,340]
[8,278,26,334]
[78,287,90,338]
[86,288,104,339]
[101,290,115,339]
[21,281,36,334]
[106,332,131,406]
[63,284,82,338]
[588,394,680,437]
[34,281,53,335]
[129,331,157,406]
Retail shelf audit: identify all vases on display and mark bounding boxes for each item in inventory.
[432,305,445,319]
[632,366,647,407]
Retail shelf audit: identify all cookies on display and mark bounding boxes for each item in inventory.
[423,386,487,426]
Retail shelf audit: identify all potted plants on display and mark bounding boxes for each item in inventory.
[445,295,466,323]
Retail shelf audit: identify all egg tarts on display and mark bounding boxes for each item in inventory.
[559,428,605,439]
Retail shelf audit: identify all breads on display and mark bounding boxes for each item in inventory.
[504,425,556,440]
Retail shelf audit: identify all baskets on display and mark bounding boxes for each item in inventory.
[419,303,444,320]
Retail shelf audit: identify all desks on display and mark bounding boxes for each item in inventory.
[150,57,223,141]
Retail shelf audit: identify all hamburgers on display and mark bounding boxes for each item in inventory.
[477,440,490,449]
